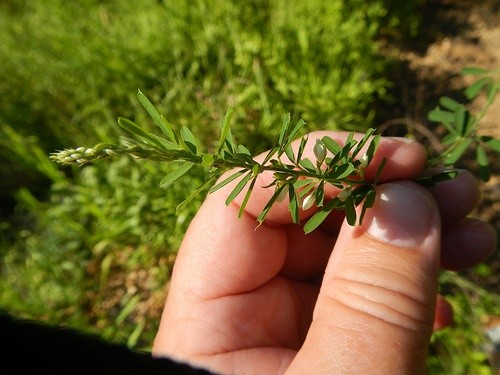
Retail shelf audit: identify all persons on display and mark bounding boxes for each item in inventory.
[0,128,494,375]
